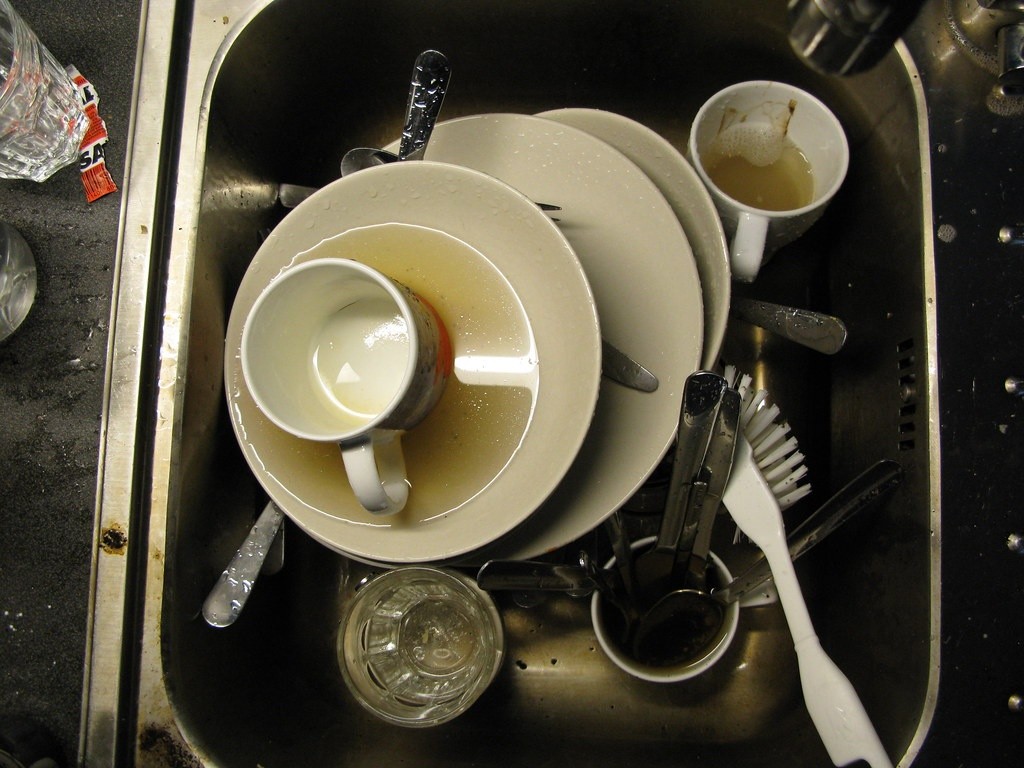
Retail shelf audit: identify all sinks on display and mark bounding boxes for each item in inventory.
[158,0,940,768]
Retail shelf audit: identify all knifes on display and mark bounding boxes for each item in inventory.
[476,371,741,650]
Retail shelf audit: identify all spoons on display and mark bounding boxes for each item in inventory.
[634,456,905,665]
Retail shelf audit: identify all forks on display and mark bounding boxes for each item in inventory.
[274,183,562,221]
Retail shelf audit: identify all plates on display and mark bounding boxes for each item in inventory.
[533,105,732,374]
[297,113,706,569]
[225,162,601,564]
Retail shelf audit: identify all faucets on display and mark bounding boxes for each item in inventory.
[786,0,1024,100]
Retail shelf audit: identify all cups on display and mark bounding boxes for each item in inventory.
[686,82,848,287]
[240,257,449,513]
[333,565,504,727]
[0,1,90,184]
[0,220,37,341]
[590,535,778,682]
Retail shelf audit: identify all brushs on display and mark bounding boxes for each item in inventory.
[706,365,894,768]
